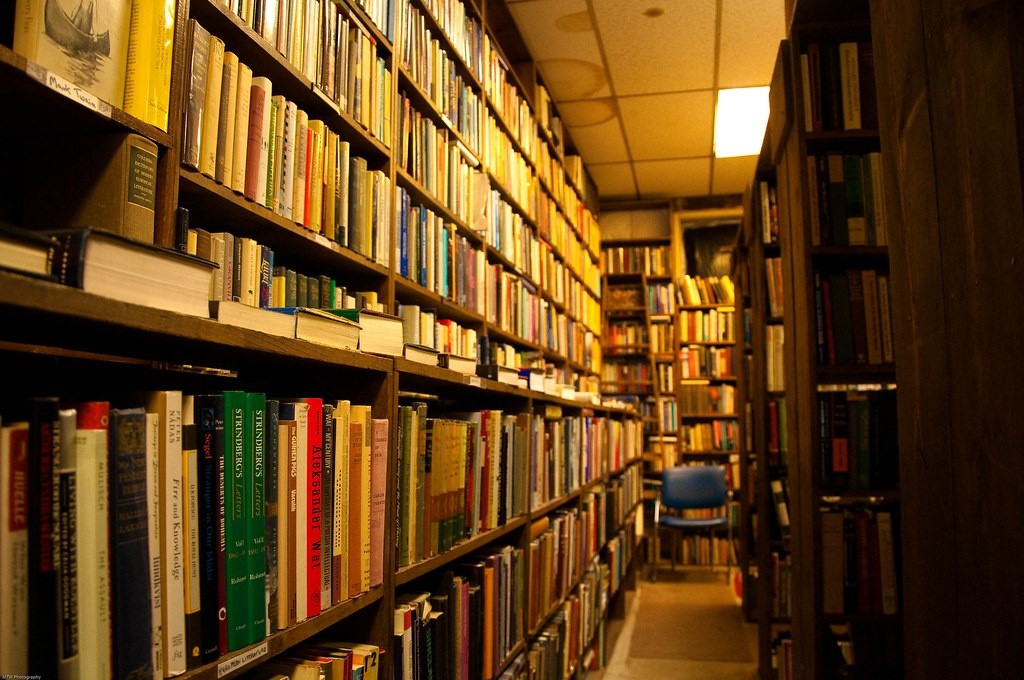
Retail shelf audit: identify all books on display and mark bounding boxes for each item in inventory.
[0,0,905,680]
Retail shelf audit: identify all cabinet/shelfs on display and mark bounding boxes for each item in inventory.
[3,2,1024,677]
